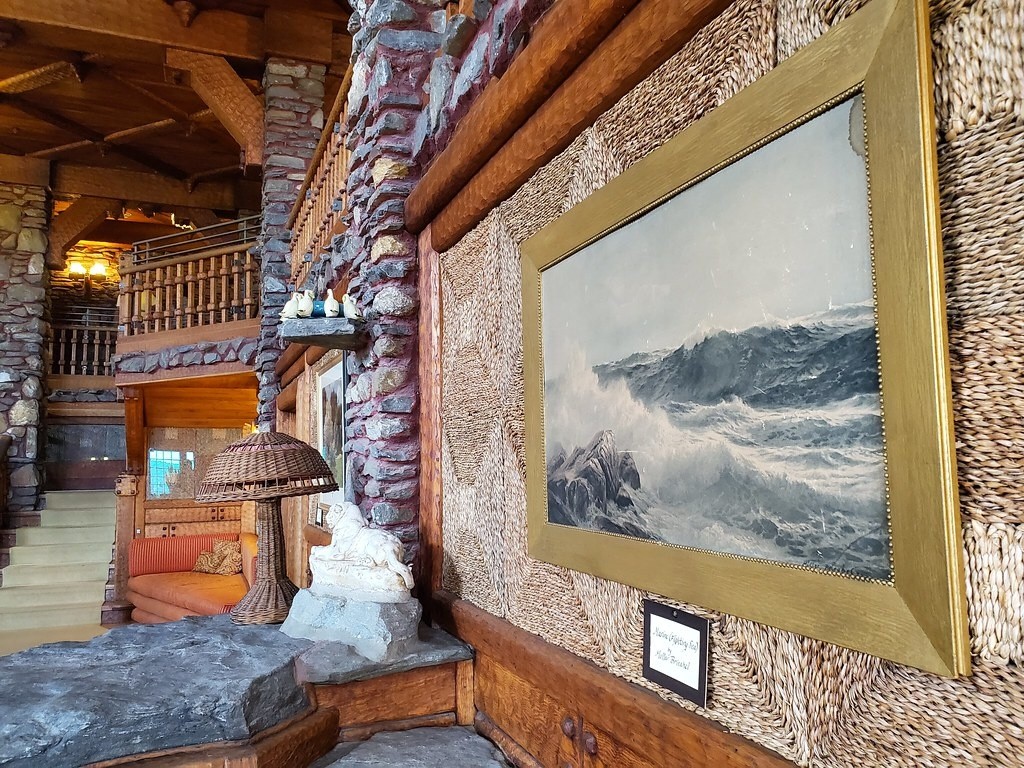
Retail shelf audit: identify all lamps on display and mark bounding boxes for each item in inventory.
[194,430,340,624]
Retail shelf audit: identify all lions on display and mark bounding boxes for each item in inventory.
[310,501,404,566]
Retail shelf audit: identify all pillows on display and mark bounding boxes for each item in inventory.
[191,536,243,577]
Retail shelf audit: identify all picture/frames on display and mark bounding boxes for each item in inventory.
[641,597,713,711]
[518,0,976,681]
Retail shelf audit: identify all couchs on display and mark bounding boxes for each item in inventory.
[126,531,258,625]
